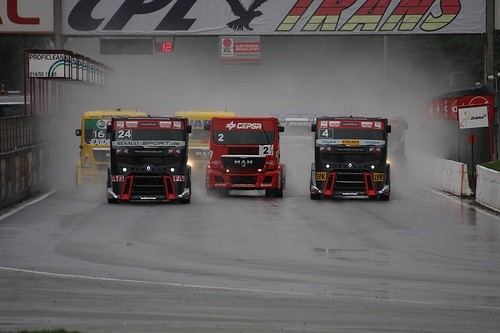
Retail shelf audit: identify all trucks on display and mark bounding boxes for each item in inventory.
[76,111,149,195]
[105,114,193,204]
[274,113,315,175]
[204,115,285,198]
[386,112,408,181]
[174,111,237,172]
[309,115,392,200]
